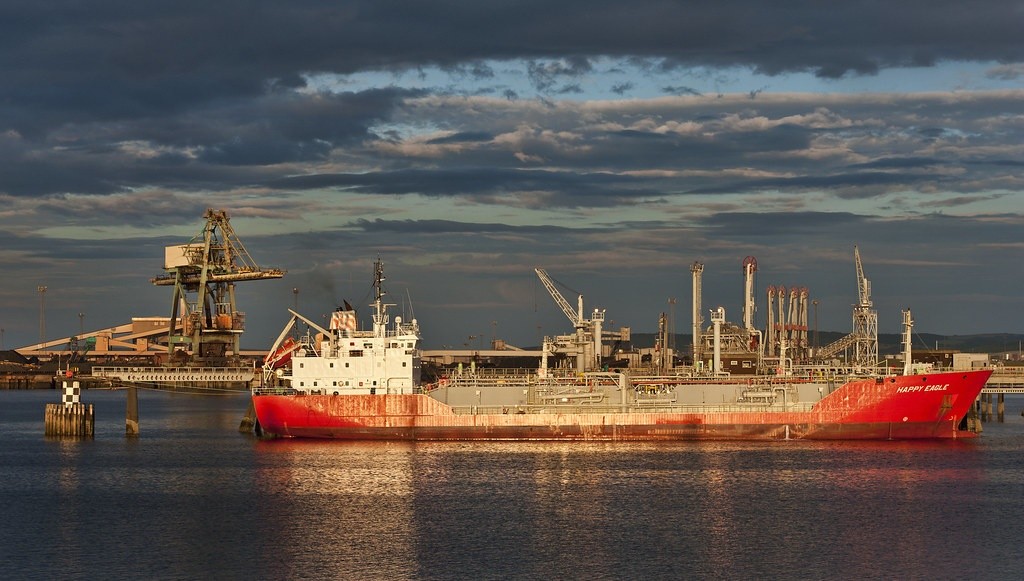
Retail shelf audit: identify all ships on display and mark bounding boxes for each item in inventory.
[251,250,995,442]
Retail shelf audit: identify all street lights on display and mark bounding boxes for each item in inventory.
[79,313,84,340]
[38,286,49,349]
[292,287,298,344]
